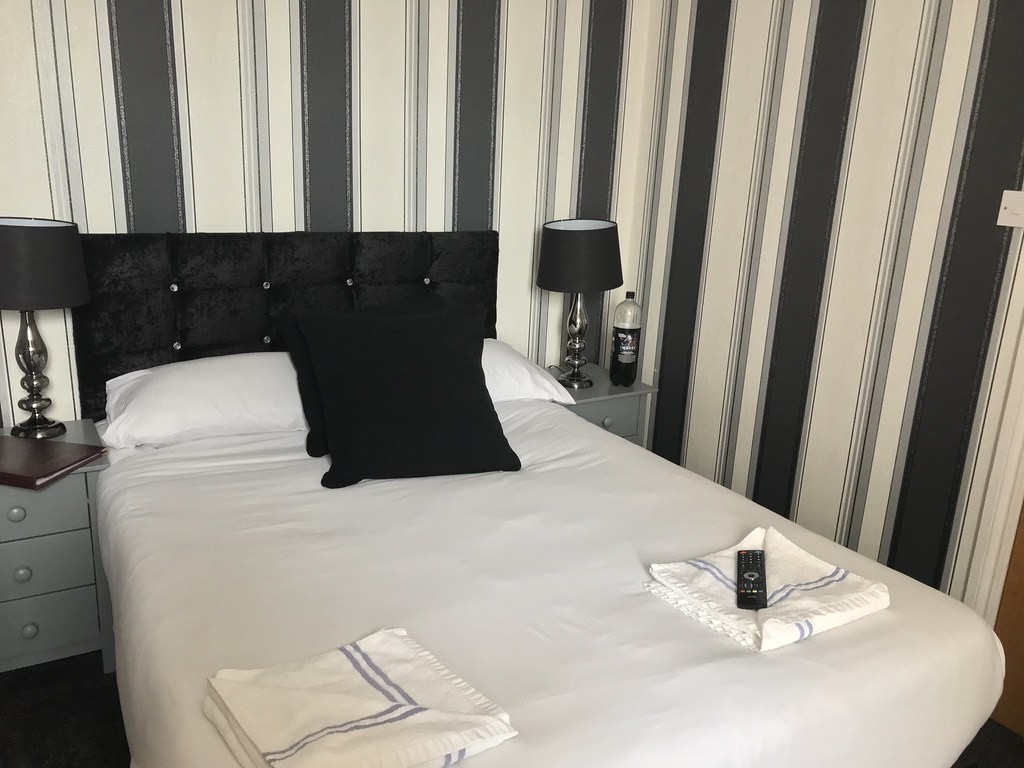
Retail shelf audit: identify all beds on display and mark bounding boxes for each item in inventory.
[61,228,1005,768]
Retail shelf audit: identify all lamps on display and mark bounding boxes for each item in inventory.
[0,216,89,440]
[536,217,623,392]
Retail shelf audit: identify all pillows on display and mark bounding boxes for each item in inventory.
[295,297,522,488]
[101,351,310,450]
[278,314,332,460]
[476,339,575,409]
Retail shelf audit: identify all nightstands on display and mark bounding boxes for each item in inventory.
[1,415,116,677]
[540,362,659,450]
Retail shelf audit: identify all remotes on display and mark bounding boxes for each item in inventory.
[737,549,768,609]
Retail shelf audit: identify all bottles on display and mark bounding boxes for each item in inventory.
[610,291,642,386]
